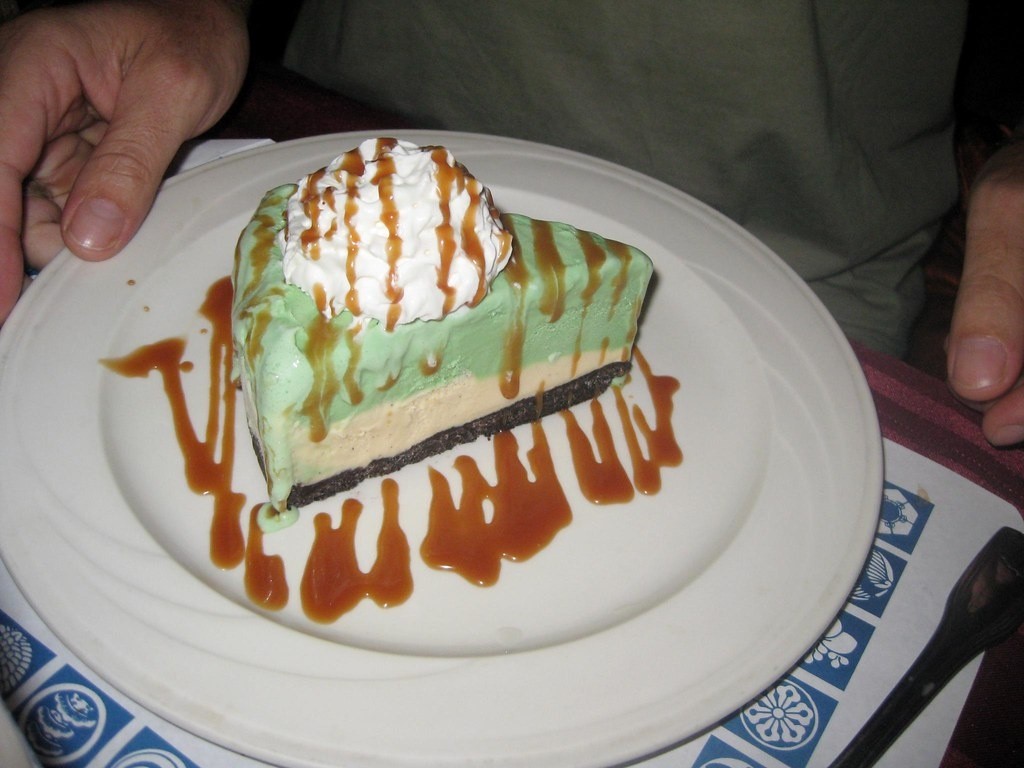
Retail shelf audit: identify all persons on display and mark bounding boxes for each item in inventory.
[0,0,1024,453]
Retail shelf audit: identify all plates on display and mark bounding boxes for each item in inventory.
[1,127,886,767]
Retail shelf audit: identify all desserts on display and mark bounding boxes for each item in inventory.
[230,138,654,532]
